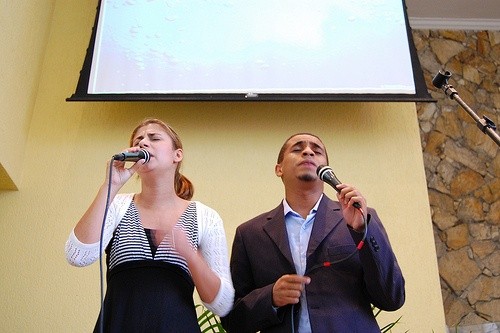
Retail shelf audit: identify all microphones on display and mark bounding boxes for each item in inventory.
[316,165,361,210]
[113,150,150,165]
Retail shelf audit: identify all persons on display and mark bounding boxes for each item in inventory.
[219,133,406,333]
[63,117,237,333]
